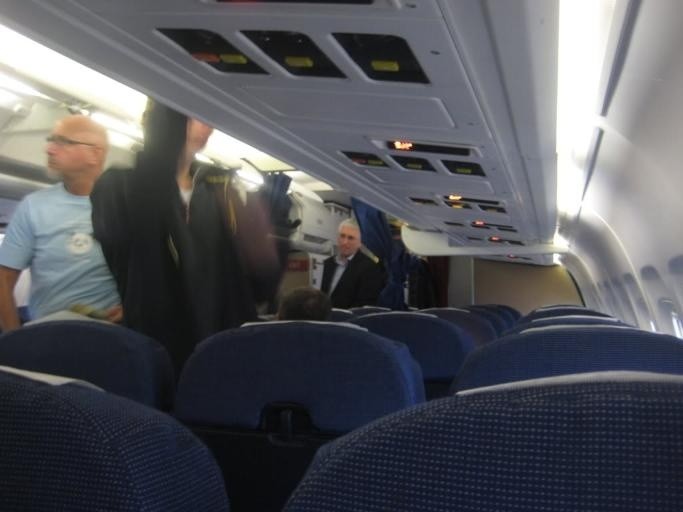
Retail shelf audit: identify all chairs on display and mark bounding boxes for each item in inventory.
[0,304,683,512]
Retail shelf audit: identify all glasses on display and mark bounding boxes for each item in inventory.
[44,133,96,149]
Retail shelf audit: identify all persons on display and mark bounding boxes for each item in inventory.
[277,286,330,323]
[90,96,258,370]
[0,113,125,335]
[320,217,378,310]
